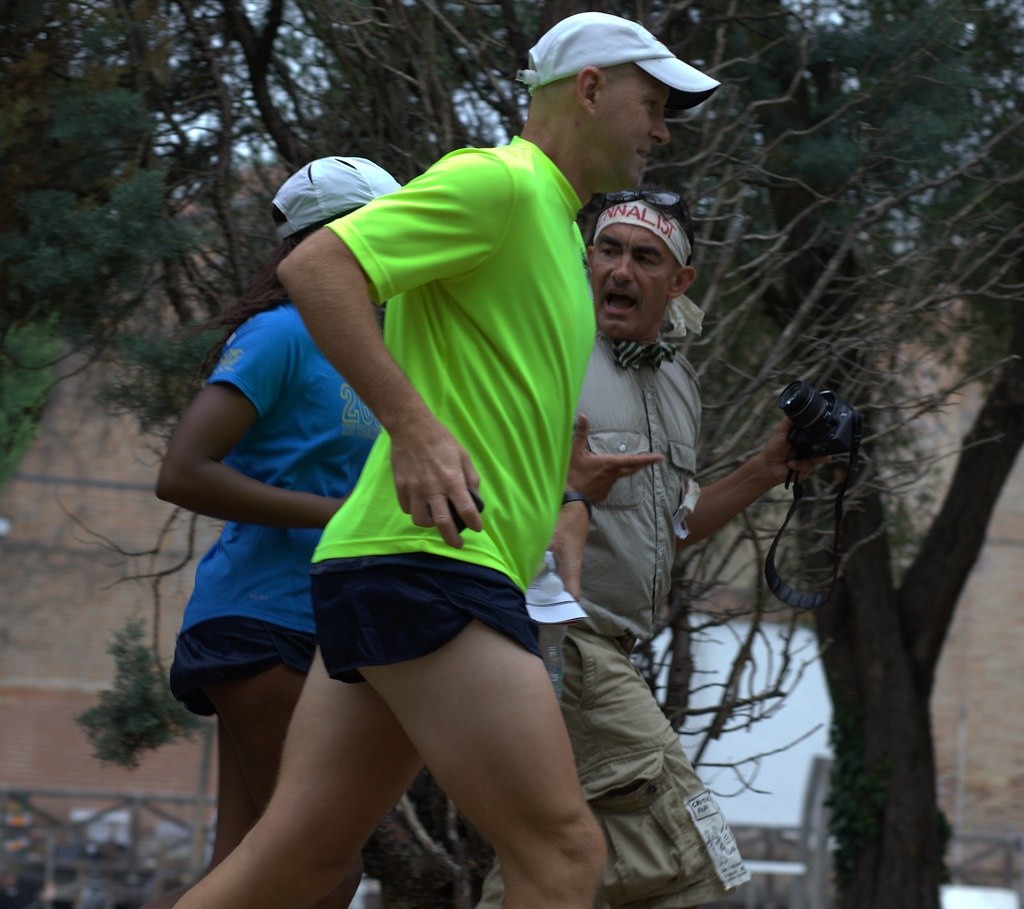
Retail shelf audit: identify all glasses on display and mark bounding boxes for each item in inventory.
[601,187,686,222]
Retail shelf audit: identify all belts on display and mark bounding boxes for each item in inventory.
[618,628,636,654]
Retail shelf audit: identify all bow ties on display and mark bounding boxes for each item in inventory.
[610,338,678,371]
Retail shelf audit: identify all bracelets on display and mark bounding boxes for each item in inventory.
[561,491,593,520]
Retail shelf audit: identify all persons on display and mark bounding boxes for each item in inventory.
[155,9,833,909]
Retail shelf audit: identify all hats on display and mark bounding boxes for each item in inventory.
[516,12,721,112]
[270,156,404,241]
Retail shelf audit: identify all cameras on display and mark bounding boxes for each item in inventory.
[780,380,869,461]
